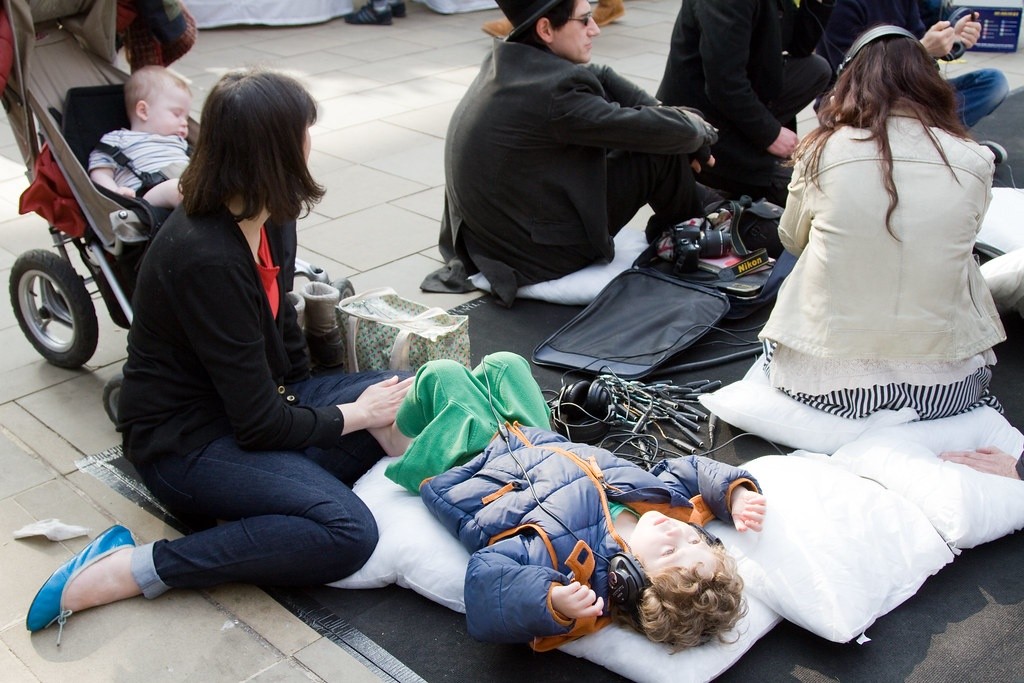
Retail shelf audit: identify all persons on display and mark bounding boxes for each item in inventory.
[935,443,1024,478]
[439,0,727,309]
[344,0,408,25]
[87,63,198,209]
[650,1,1009,418]
[363,350,764,655]
[24,70,425,632]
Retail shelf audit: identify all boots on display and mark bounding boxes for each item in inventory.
[301,281,345,378]
[285,292,315,376]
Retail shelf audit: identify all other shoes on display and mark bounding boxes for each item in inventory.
[481,17,514,37]
[389,3,406,18]
[344,2,392,25]
[592,0,624,26]
[646,216,671,244]
[25,525,135,647]
[978,140,1008,165]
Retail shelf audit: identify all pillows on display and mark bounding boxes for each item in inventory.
[467,230,648,307]
[975,186,1024,317]
[323,354,1023,683]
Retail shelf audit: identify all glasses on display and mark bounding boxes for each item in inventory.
[568,11,593,27]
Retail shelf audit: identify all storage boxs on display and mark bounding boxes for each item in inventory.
[943,0,1024,52]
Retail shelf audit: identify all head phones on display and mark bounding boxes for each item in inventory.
[607,523,726,613]
[551,377,614,443]
[835,25,941,79]
[941,6,976,62]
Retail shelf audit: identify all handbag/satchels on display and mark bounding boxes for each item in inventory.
[337,287,471,372]
[532,194,798,381]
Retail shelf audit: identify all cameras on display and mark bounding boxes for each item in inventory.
[670,224,733,273]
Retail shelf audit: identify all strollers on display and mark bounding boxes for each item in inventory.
[0,0,357,425]
